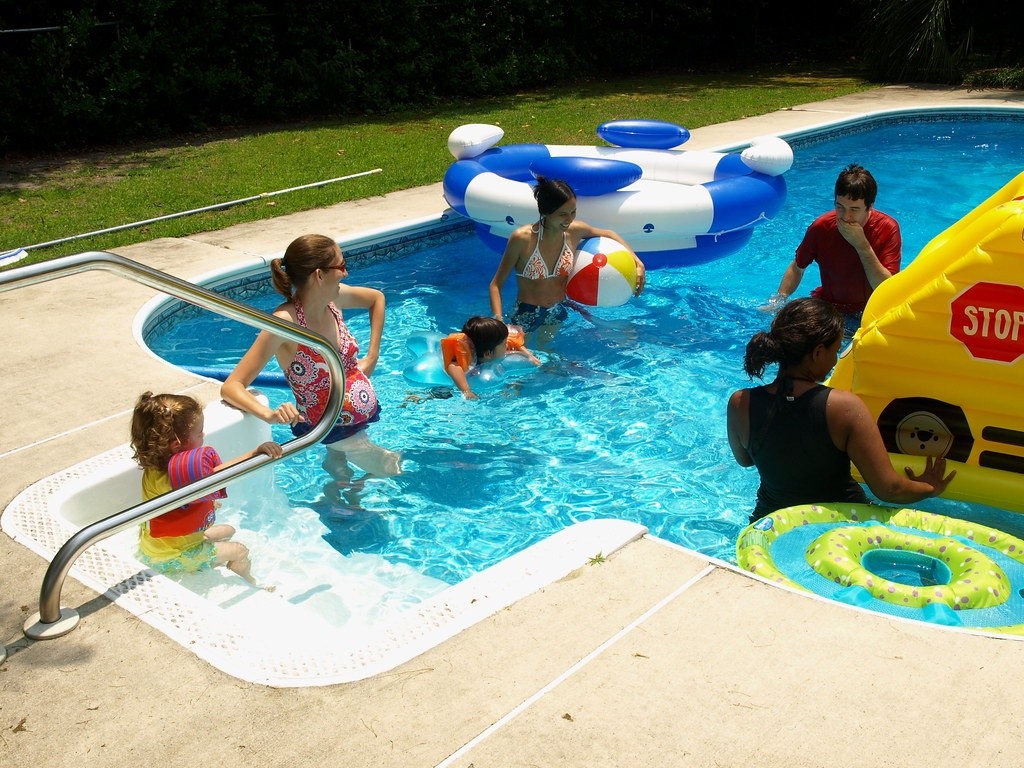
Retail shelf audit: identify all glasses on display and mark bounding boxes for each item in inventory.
[309,262,347,277]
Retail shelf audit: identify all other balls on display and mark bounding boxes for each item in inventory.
[566,236,638,305]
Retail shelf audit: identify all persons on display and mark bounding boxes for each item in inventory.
[220,234,386,446]
[131,392,246,575]
[442,317,541,399]
[778,163,901,320]
[727,298,957,523]
[489,178,646,333]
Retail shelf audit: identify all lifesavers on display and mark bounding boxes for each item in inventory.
[167,445,227,503]
[402,329,535,389]
[440,333,477,374]
[505,323,525,350]
[738,487,1024,640]
